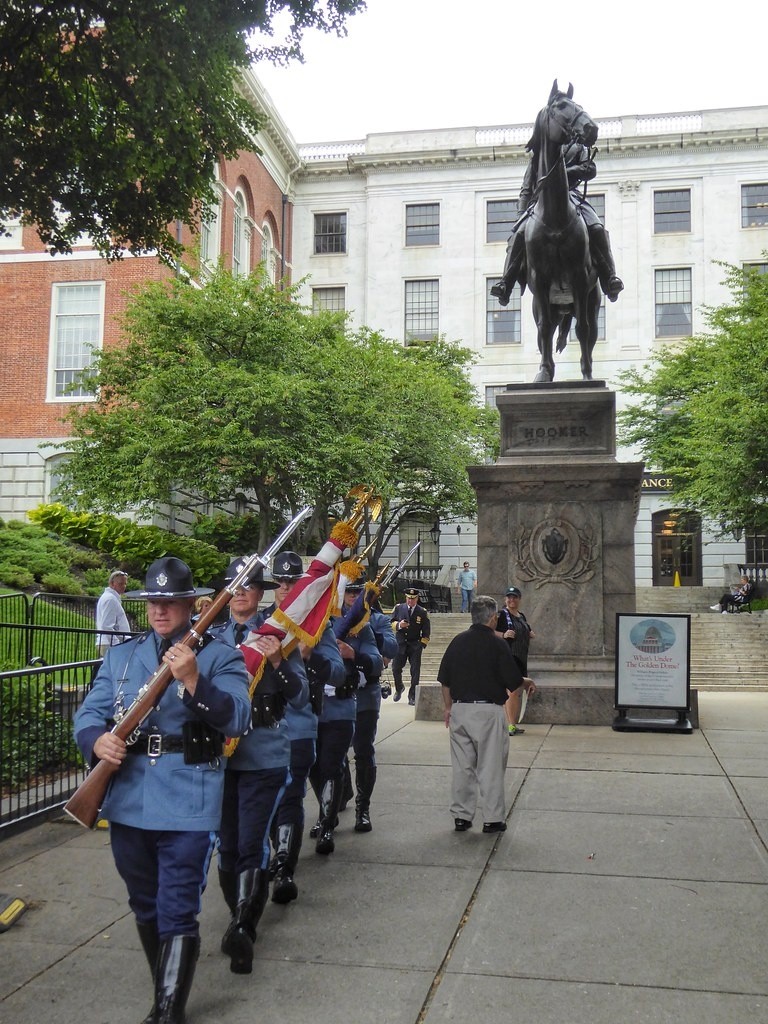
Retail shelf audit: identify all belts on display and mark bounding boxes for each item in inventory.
[452,699,493,703]
[126,734,184,757]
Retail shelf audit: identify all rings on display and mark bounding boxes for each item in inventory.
[170,656,174,659]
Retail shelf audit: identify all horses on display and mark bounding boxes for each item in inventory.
[523,78,602,383]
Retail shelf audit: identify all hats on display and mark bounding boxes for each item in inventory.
[271,551,303,579]
[124,557,216,598]
[223,556,281,590]
[345,571,367,591]
[505,586,521,597]
[403,588,420,598]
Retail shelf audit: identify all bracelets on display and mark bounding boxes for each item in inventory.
[503,633,504,638]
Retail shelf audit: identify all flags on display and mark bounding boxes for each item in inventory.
[334,581,380,641]
[330,561,366,617]
[225,523,357,755]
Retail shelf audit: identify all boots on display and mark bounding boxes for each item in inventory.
[135,765,379,1024]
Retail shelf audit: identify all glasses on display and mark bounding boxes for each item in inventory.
[464,566,469,568]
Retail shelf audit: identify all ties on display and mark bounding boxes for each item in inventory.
[235,623,248,645]
[158,639,172,666]
[408,607,412,617]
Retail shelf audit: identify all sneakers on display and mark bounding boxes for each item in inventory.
[508,724,516,736]
[515,728,525,733]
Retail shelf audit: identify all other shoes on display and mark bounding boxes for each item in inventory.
[454,818,472,832]
[722,611,728,614]
[483,822,507,833]
[461,611,465,613]
[393,686,405,701]
[710,605,719,611]
[408,694,415,705]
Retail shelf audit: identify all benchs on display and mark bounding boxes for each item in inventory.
[417,591,449,613]
[727,586,755,614]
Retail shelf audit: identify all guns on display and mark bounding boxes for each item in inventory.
[369,541,422,609]
[62,505,312,831]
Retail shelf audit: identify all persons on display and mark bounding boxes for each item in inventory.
[457,562,477,613]
[490,143,625,306]
[710,575,751,613]
[262,552,346,905]
[309,617,384,855]
[95,571,131,655]
[192,596,213,620]
[210,558,308,975]
[338,578,398,833]
[494,586,536,736]
[390,588,430,705]
[437,596,537,833]
[73,558,252,1015]
